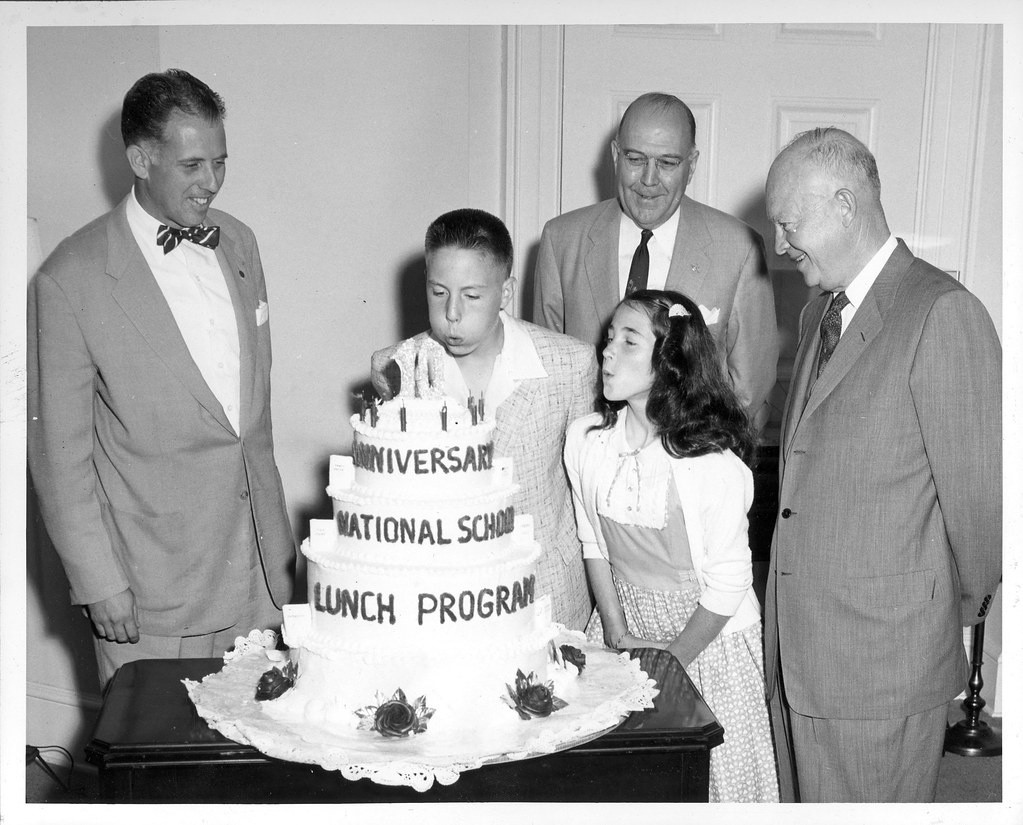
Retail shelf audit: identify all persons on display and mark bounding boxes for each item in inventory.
[26,69,297,692]
[371,93,1002,803]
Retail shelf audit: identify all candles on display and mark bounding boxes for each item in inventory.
[360,398,366,421]
[370,398,379,428]
[468,388,474,408]
[400,398,407,432]
[478,389,485,421]
[472,399,478,426]
[442,400,448,431]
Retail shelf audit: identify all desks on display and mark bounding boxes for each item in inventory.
[82,647,725,803]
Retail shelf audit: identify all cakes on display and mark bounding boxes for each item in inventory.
[256,341,586,739]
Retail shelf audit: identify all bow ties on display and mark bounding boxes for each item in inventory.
[156,219,220,254]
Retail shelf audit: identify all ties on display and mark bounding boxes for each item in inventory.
[816,291,850,379]
[623,229,653,299]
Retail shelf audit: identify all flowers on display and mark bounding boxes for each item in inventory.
[499,668,570,720]
[256,660,301,701]
[549,639,587,676]
[355,687,437,738]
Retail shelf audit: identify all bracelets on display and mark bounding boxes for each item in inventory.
[612,630,632,649]
